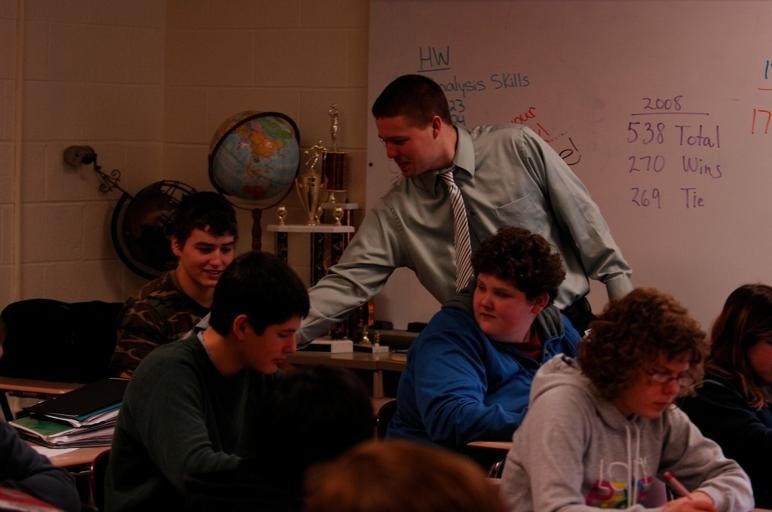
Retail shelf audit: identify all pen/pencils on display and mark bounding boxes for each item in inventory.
[662,471,690,498]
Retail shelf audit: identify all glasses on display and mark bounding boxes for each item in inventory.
[638,366,697,388]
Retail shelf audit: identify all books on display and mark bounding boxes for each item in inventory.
[7,382,126,448]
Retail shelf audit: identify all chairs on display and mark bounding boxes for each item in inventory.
[86,450,116,512]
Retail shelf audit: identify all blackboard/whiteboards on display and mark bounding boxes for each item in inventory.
[367,0,771,338]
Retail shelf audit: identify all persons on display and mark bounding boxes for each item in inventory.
[279,66,635,355]
[109,188,240,381]
[678,279,772,511]
[493,286,756,511]
[381,224,585,477]
[96,250,325,512]
[0,418,82,512]
[301,436,509,512]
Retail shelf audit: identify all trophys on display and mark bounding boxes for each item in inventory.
[294,101,351,227]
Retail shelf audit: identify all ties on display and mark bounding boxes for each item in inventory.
[440,171,474,290]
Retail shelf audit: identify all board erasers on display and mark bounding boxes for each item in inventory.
[408,322,427,332]
[375,320,393,330]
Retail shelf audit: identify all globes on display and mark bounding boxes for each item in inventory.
[207,109,303,254]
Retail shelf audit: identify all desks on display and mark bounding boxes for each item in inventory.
[16,349,515,469]
